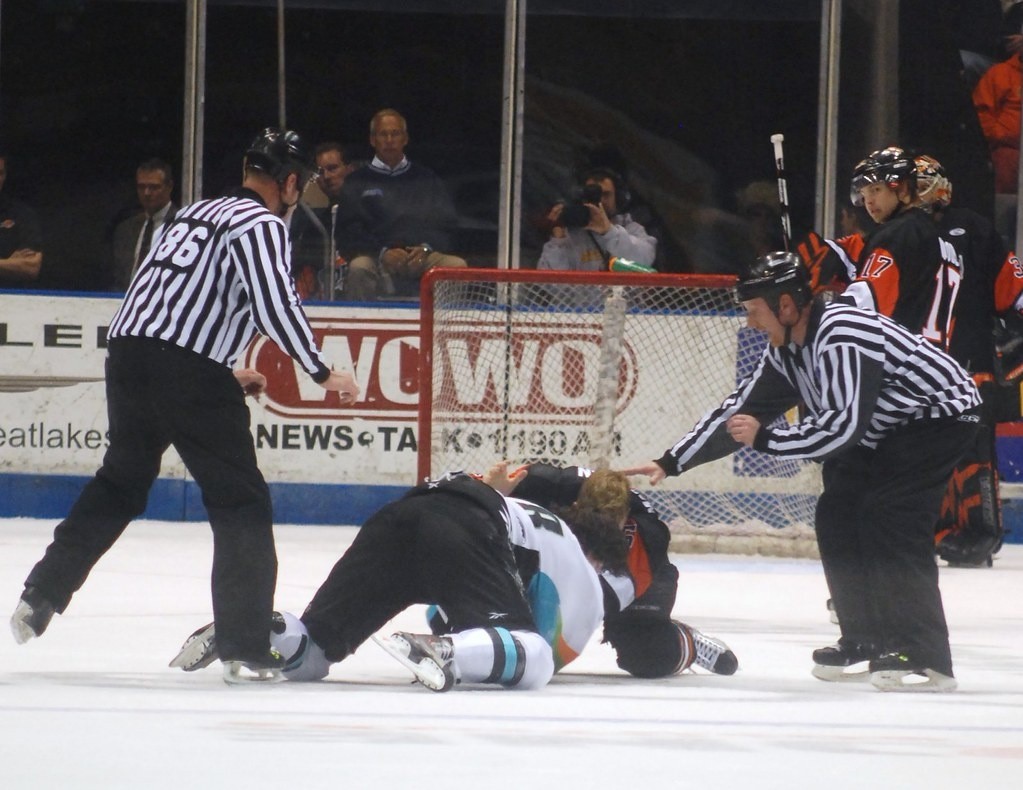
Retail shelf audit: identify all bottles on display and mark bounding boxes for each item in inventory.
[331,250,347,290]
[607,257,656,278]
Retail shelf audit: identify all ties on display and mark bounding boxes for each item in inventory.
[136,220,154,270]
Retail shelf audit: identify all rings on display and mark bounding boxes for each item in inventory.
[418,259,422,265]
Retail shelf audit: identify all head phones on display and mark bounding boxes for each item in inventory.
[614,174,632,210]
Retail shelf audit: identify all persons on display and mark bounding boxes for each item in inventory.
[534,165,658,310]
[941,0,1023,193]
[168,460,738,693]
[11,127,360,683]
[612,251,984,693]
[295,143,357,301]
[111,158,179,292]
[797,146,966,354]
[0,151,46,289]
[334,109,469,302]
[912,154,1023,569]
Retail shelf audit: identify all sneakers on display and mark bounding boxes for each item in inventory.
[869,649,959,693]
[9,584,57,645]
[220,645,288,684]
[672,620,741,676]
[168,610,287,671]
[812,635,884,683]
[370,630,462,693]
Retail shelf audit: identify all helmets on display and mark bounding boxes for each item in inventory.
[850,147,918,193]
[914,155,952,213]
[246,127,314,190]
[731,251,809,311]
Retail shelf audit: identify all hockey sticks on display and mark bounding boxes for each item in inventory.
[770,131,795,252]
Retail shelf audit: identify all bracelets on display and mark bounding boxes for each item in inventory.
[420,246,431,256]
